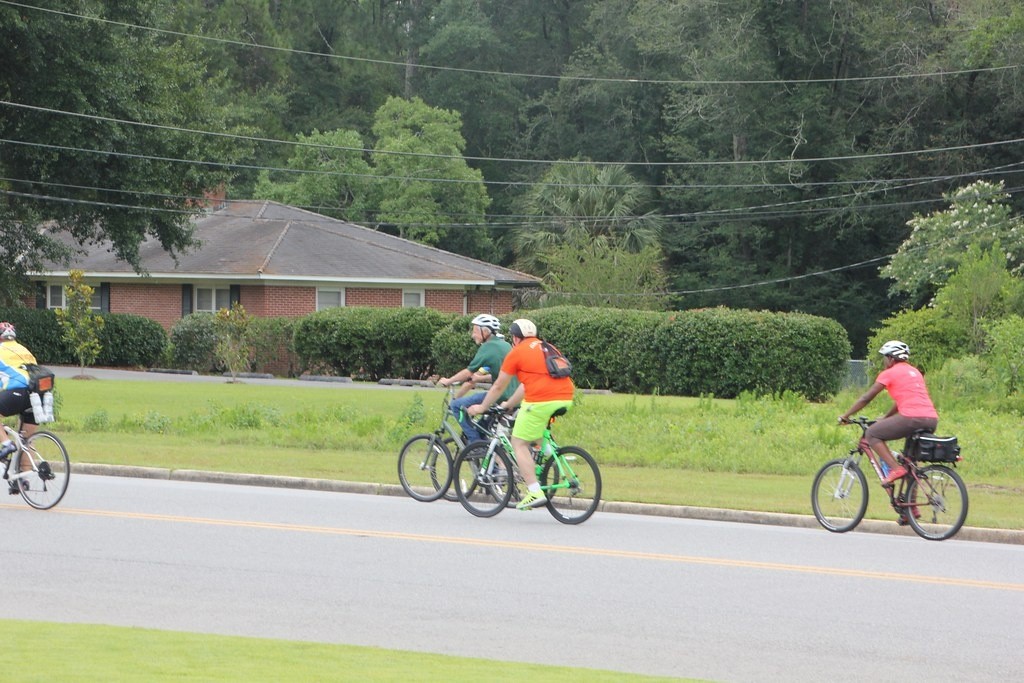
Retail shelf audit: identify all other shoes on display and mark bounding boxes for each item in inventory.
[463,447,487,459]
[485,467,500,496]
[0,441,17,459]
[8,478,30,494]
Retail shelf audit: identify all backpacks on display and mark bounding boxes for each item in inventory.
[0,344,54,404]
[516,339,572,379]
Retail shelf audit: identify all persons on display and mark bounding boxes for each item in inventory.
[438,314,575,510]
[838,340,939,526]
[0,322,50,490]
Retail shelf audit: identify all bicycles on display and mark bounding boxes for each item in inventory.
[397,380,559,510]
[811,417,970,541]
[0,410,71,510]
[452,406,602,525]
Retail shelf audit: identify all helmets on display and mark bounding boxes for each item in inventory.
[879,341,910,360]
[508,319,537,339]
[471,314,500,329]
[0,322,16,339]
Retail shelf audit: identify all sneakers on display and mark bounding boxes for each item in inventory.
[879,466,907,486]
[515,489,548,509]
[542,453,560,462]
[896,506,921,524]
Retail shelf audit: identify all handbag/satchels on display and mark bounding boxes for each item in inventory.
[915,432,960,462]
